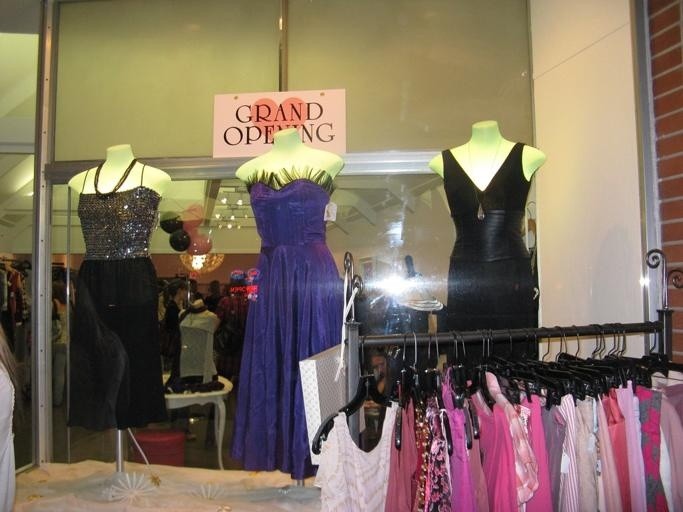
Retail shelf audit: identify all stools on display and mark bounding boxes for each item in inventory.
[129,429,187,468]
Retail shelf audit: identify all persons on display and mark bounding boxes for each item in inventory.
[157,278,239,373]
[64,142,171,433]
[0,327,19,512]
[429,118,546,364]
[175,299,222,390]
[228,127,346,478]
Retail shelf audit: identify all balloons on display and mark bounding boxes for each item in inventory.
[187,234,213,255]
[169,230,191,252]
[183,224,198,234]
[181,204,205,229]
[159,211,183,233]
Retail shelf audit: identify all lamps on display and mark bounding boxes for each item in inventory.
[405,255,422,279]
[180,253,225,275]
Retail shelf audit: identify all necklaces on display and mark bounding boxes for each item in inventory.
[93,157,138,199]
[474,188,487,223]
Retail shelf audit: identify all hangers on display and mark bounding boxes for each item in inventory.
[310,315,683,454]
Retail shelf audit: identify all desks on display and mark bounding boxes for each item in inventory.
[126,373,234,470]
